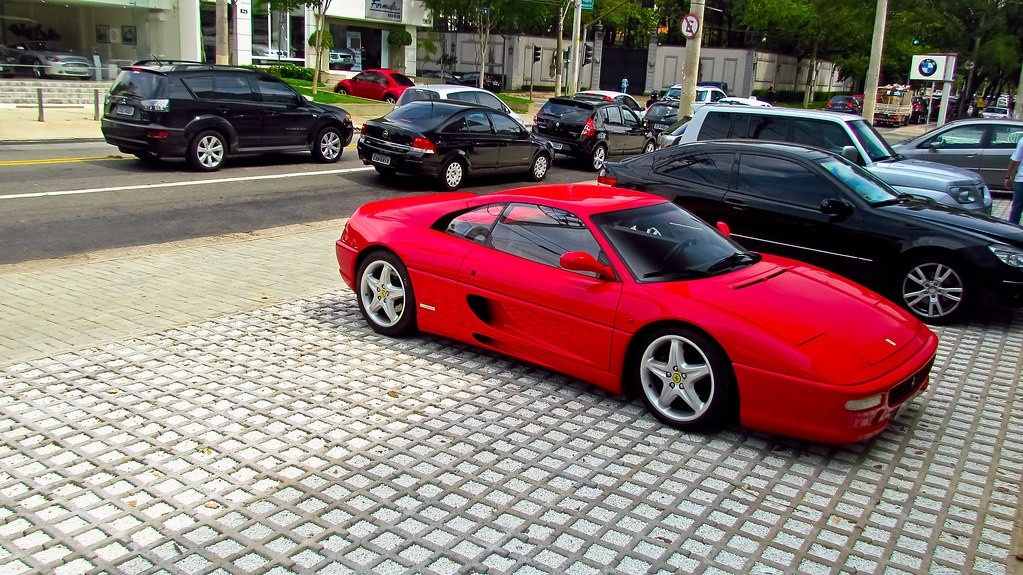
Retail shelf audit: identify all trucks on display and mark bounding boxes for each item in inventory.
[875,85,915,127]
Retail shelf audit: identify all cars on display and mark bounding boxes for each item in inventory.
[890,119,1023,191]
[354,98,556,192]
[0,43,17,77]
[910,92,1014,119]
[600,138,1023,330]
[424,72,455,80]
[638,99,697,140]
[335,68,416,107]
[827,96,861,116]
[530,97,659,171]
[653,115,695,150]
[572,90,647,124]
[332,181,941,446]
[717,96,773,109]
[445,71,501,96]
[328,47,356,70]
[251,42,293,65]
[396,84,526,132]
[7,39,95,79]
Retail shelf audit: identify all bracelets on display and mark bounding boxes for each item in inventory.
[1005,176,1013,179]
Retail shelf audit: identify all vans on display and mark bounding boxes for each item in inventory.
[700,81,728,96]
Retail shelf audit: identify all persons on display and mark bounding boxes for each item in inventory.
[975,96,987,118]
[768,85,777,103]
[1004,137,1023,223]
[621,77,628,93]
[646,93,660,109]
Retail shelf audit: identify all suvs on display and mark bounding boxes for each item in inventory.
[662,84,728,106]
[676,101,996,218]
[97,59,353,172]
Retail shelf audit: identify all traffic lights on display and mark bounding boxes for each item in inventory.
[912,36,922,47]
[580,44,594,66]
[532,43,542,65]
